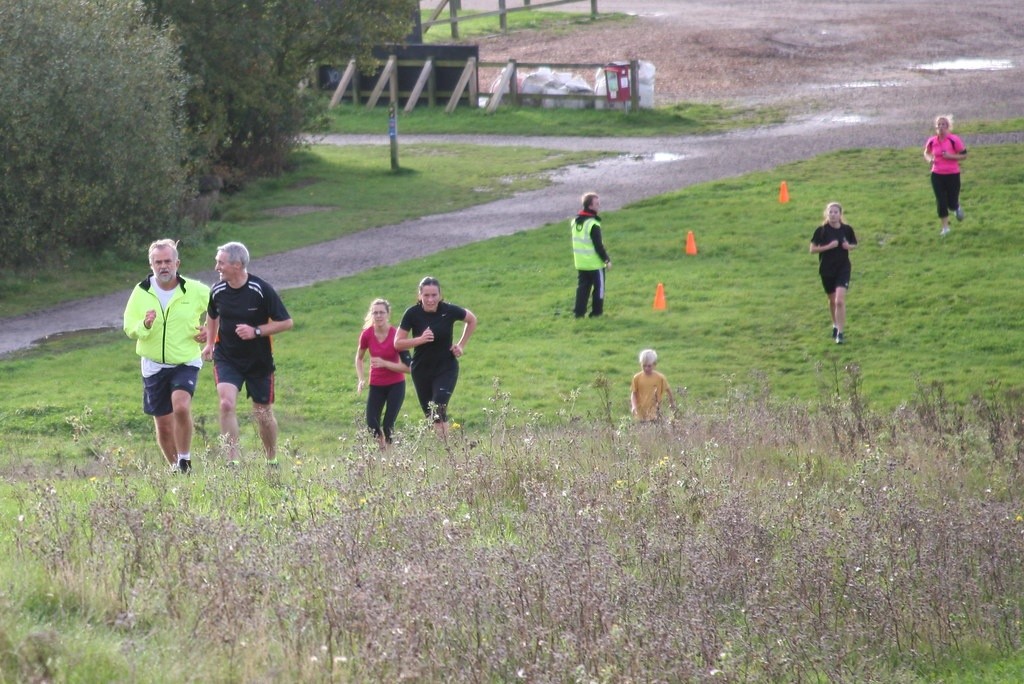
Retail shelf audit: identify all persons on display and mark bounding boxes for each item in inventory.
[355,301,412,454]
[394,277,477,440]
[631,350,675,425]
[810,203,857,343]
[924,116,968,236]
[202,242,293,474]
[571,193,611,317]
[124,239,210,476]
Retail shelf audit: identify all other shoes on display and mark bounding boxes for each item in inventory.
[939,228,950,236]
[954,204,964,221]
[178,459,192,475]
[836,333,843,344]
[832,327,837,338]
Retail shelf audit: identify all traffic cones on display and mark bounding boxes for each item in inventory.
[685,231,698,254]
[653,283,667,309]
[778,181,791,204]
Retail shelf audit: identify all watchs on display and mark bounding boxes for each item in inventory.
[254,326,261,338]
